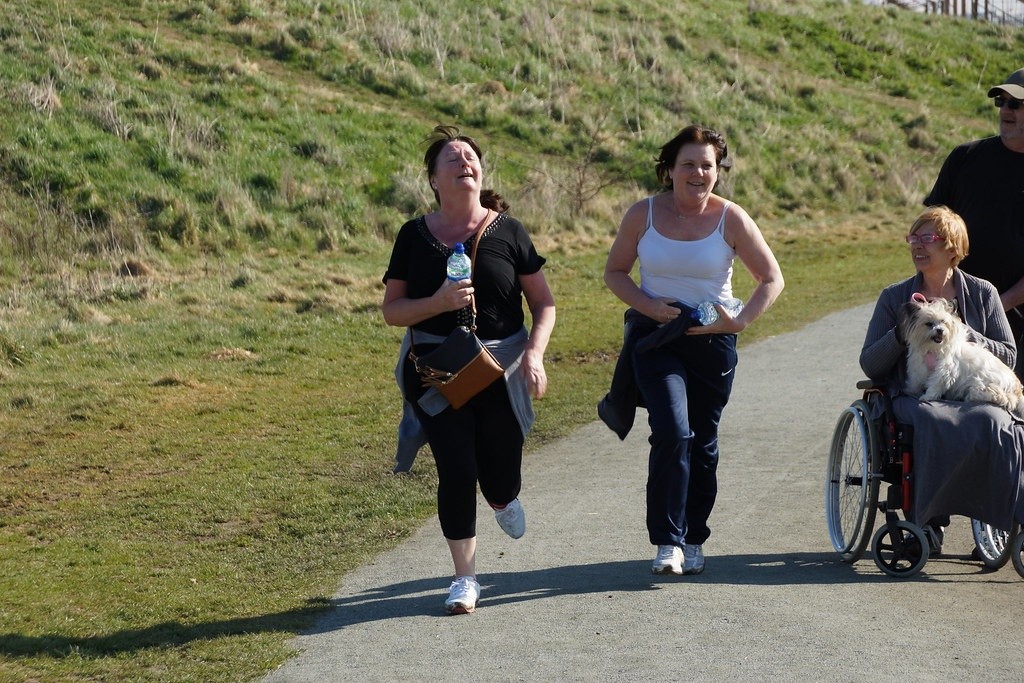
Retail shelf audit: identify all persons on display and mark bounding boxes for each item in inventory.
[860,207,1017,557]
[603,126,783,574]
[382,125,556,613]
[901,67,1024,558]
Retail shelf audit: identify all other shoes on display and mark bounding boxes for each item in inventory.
[969,544,985,564]
[902,530,944,559]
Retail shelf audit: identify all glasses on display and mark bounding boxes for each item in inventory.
[995,95,1024,110]
[906,234,947,244]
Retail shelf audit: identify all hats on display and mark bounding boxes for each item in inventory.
[988,67,1024,100]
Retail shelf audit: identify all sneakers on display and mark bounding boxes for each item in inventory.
[444,576,481,614]
[682,544,705,574]
[481,490,526,539]
[651,543,685,575]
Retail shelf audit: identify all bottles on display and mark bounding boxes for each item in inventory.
[446,242,472,288]
[689,298,744,326]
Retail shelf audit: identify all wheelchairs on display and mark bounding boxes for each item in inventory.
[825,380,1024,579]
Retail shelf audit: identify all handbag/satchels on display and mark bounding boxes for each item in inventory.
[439,355,507,410]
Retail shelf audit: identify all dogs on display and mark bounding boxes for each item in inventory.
[898,296,1024,418]
